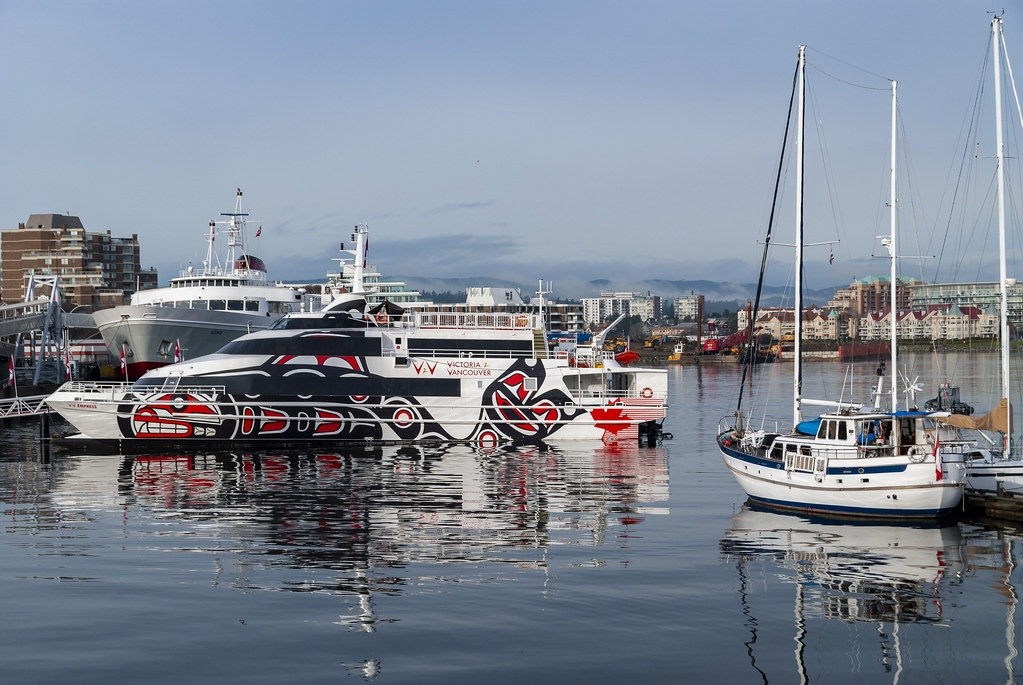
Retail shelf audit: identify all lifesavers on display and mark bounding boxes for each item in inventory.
[908,445,925,462]
[642,387,653,397]
[377,312,387,324]
[518,314,528,325]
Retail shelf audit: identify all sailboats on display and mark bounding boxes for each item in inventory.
[714,9,1023,519]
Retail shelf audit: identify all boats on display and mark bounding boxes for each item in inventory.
[42,185,675,455]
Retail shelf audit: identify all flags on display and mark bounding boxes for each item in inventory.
[173,339,181,363]
[255,225,262,237]
[64,351,71,380]
[361,236,369,269]
[931,431,946,482]
[9,357,15,386]
[119,345,126,374]
